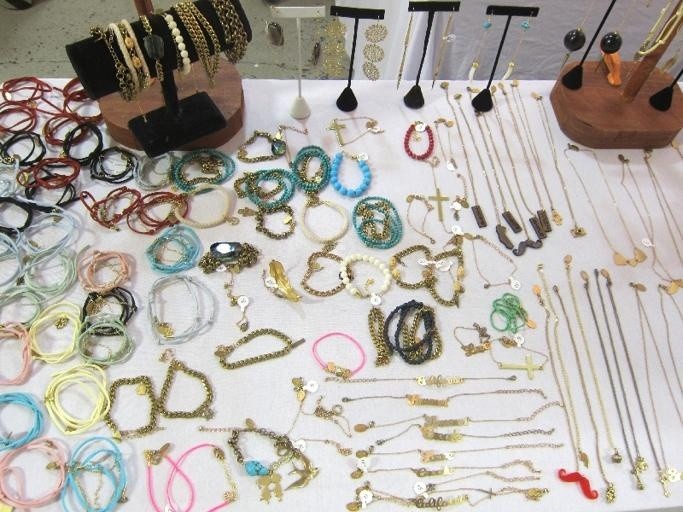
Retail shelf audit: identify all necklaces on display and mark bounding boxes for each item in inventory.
[437,74,683,512]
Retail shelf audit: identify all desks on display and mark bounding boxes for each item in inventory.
[0,78,683,512]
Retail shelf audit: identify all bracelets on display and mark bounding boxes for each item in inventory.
[0,79,565,512]
[88,1,247,100]
